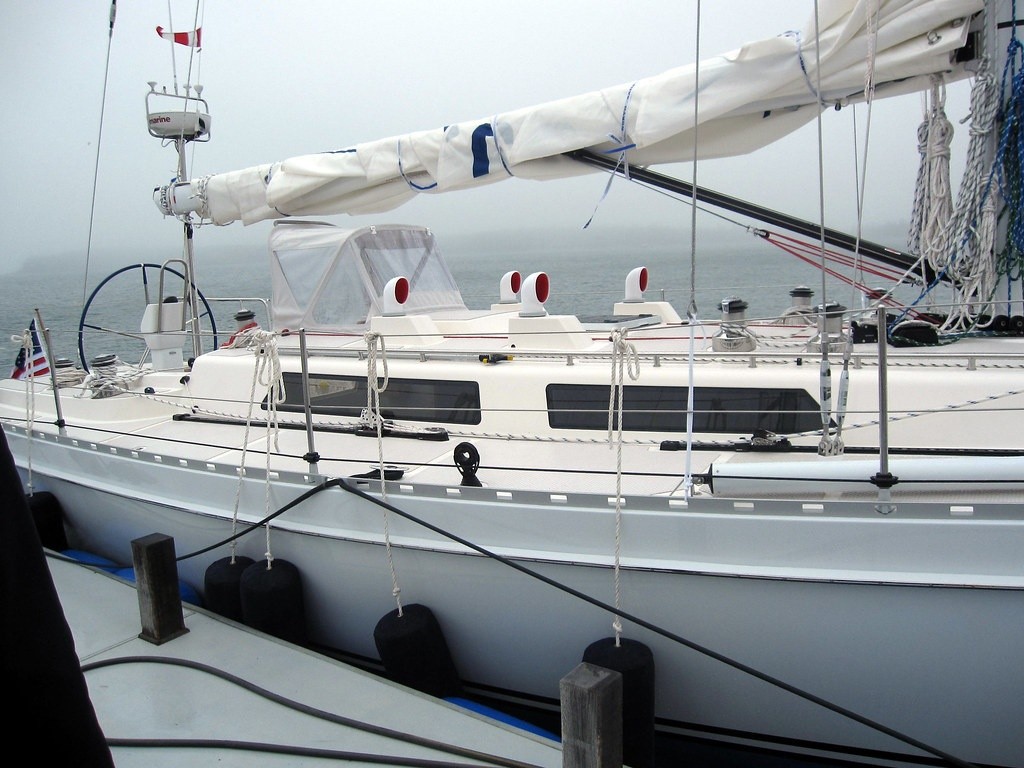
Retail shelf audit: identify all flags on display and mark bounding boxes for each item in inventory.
[155,26,201,47]
[11,319,51,380]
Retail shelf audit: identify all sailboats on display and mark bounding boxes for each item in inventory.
[0,1,1024,767]
